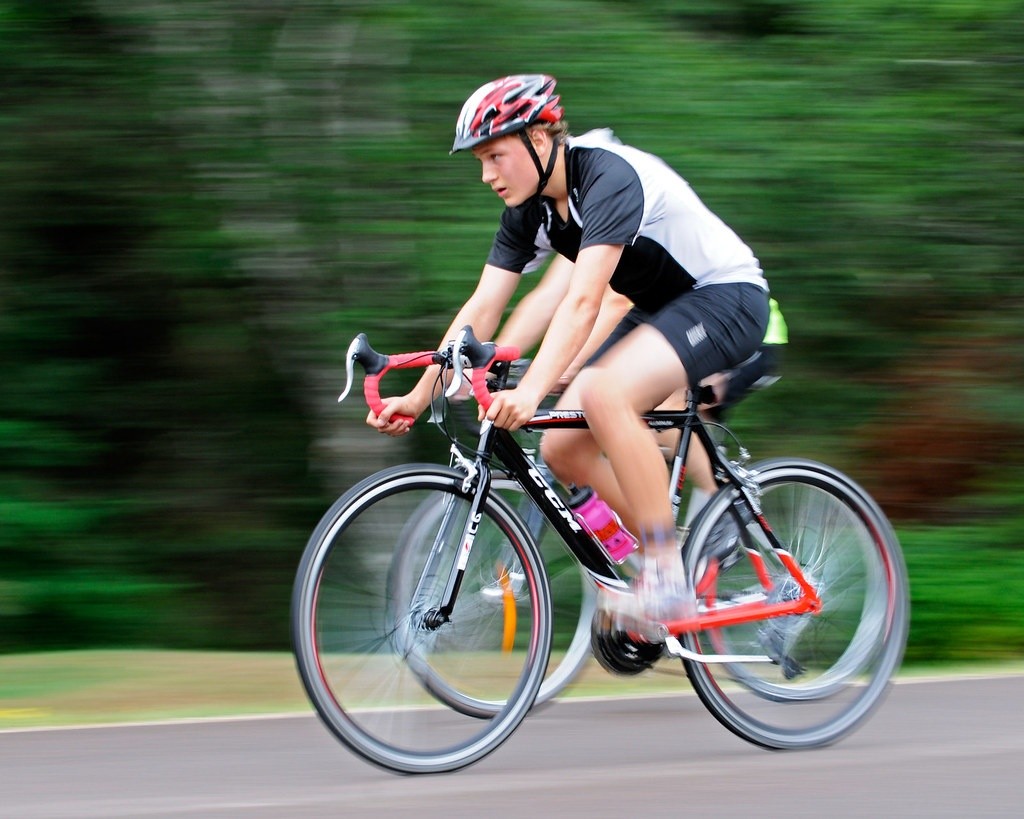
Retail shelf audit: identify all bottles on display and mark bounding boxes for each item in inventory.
[565,483,635,560]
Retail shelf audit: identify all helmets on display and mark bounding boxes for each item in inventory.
[449,73,565,153]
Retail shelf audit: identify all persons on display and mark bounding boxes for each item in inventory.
[365,74,788,623]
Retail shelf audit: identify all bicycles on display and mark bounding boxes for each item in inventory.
[289,324,913,779]
